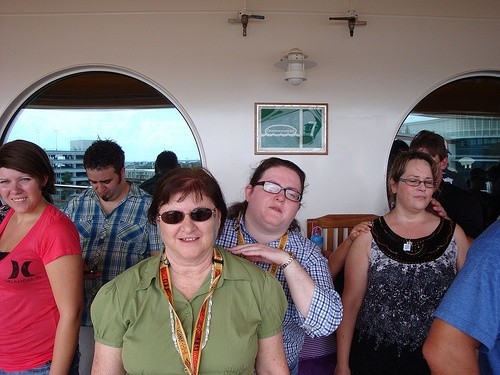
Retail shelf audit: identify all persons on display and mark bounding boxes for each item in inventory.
[331,150,471,375]
[62,140,165,375]
[420,218,500,375]
[154,150,179,176]
[299,220,376,375]
[0,138,86,375]
[409,129,485,245]
[387,141,500,239]
[88,168,292,374]
[213,154,345,375]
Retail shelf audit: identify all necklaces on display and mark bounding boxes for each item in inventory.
[392,211,424,252]
[169,264,216,353]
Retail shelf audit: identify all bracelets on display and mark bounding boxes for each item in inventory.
[277,252,298,271]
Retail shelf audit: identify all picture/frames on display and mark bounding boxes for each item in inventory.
[254,102,329,155]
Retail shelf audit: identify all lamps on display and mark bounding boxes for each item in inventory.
[274,48,318,85]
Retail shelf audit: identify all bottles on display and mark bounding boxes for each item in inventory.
[311,226,323,250]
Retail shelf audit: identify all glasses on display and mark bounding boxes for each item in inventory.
[255,181,302,202]
[158,207,216,224]
[397,178,437,188]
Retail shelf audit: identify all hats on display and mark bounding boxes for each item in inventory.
[410,130,452,154]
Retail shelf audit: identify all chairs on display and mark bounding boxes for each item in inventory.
[307,213,380,260]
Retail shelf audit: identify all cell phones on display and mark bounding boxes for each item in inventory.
[83,270,96,275]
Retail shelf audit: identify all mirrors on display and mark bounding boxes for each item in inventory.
[0,64,208,215]
[386,68,500,240]
